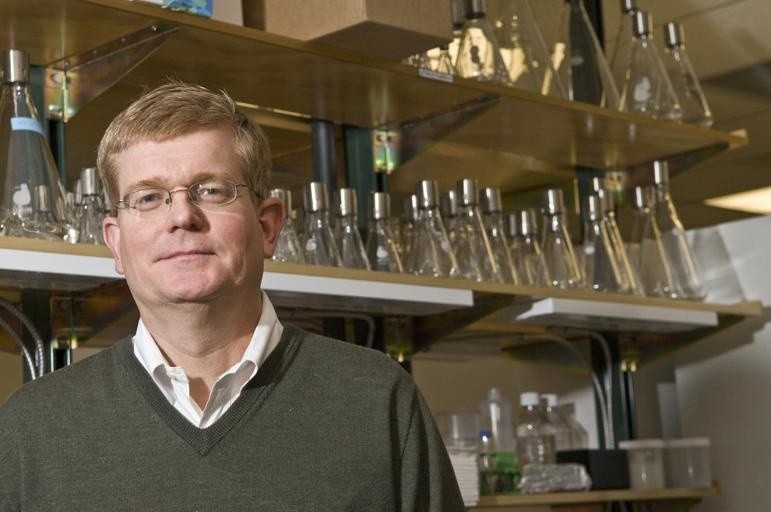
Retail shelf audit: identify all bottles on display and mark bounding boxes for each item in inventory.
[77,160,707,303]
[453,0,712,127]
[1,48,80,244]
[476,388,579,494]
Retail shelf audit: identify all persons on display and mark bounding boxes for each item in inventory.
[2,70,466,511]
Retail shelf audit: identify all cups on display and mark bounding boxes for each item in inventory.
[666,438,712,487]
[619,439,666,489]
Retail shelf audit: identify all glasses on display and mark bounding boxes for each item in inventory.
[114,177,250,208]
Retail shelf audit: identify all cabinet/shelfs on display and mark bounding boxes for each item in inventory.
[2,2,771,510]
